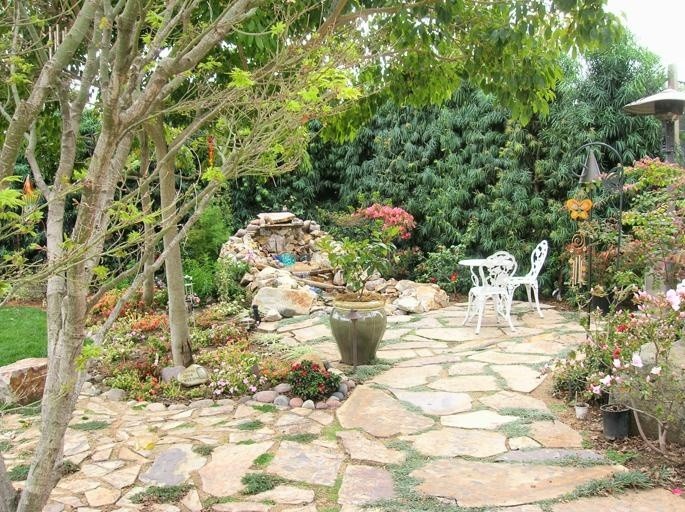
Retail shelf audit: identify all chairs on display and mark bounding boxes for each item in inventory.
[509,240,548,319]
[463,251,517,334]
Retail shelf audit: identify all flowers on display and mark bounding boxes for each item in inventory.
[314,202,417,301]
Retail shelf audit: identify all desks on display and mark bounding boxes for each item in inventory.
[458,259,513,323]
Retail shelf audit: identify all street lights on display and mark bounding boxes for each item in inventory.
[569,141,623,341]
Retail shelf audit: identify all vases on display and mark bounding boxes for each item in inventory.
[575,402,589,420]
[331,291,387,367]
[600,403,630,441]
